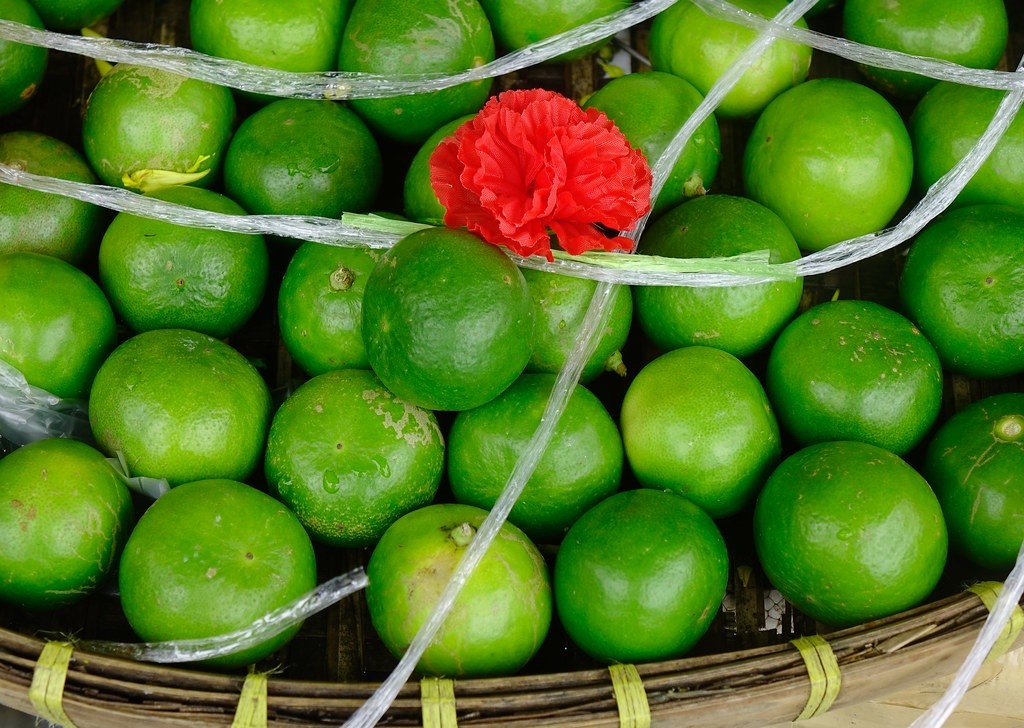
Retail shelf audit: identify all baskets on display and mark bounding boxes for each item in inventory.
[1,0,1024,728]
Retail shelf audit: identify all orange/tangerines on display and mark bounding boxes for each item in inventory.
[0,0,1024,679]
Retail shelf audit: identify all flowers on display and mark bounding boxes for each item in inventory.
[431,79,654,276]
